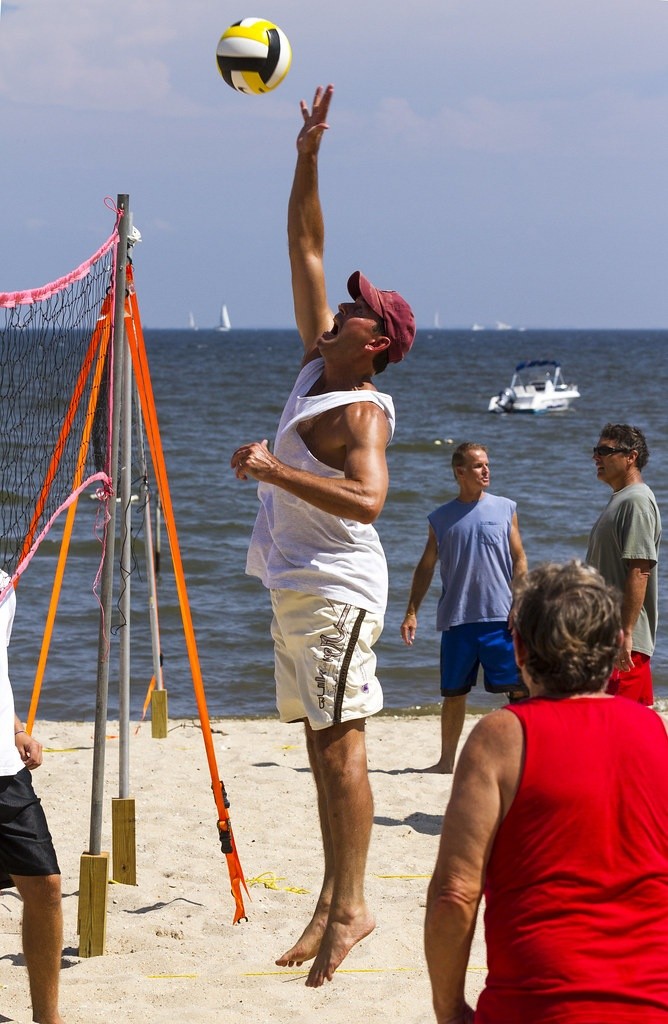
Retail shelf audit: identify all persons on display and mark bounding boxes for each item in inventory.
[586,423,662,708]
[400,442,530,774]
[0,570,66,1023]
[425,559,668,1024]
[231,85,415,989]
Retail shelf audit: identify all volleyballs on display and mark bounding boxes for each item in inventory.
[216,16,294,96]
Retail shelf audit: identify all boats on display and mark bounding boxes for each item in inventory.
[486,360,582,415]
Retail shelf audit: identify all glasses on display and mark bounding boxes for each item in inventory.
[593,446,632,456]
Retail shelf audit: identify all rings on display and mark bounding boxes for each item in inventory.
[239,460,243,467]
[400,635,404,638]
[621,660,625,662]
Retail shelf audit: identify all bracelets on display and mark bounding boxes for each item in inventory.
[15,731,28,735]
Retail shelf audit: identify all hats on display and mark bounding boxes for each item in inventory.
[347,271,416,362]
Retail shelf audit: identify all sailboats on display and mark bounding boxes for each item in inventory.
[214,304,240,336]
